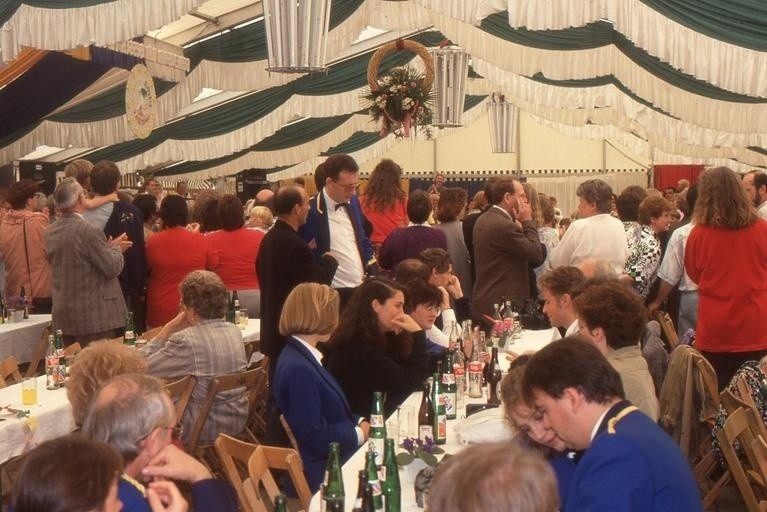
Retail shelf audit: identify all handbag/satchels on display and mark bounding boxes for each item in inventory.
[31,297,52,314]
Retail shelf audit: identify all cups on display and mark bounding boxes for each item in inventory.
[7,308,25,323]
[234,308,249,330]
[513,314,522,339]
[21,377,37,405]
[134,340,148,348]
[397,406,416,445]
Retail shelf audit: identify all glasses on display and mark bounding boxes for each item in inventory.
[161,423,185,439]
[421,300,442,317]
[35,194,40,199]
[333,179,361,191]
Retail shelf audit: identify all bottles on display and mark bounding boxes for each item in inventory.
[226,290,241,323]
[123,311,136,345]
[45,329,65,390]
[19,287,29,319]
[273,392,401,512]
[417,300,514,447]
[0,292,4,324]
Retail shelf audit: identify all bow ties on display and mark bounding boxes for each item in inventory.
[334,202,349,211]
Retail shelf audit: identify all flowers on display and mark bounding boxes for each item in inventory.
[359,65,439,141]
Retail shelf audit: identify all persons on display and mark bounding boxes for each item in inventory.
[140,270,250,445]
[273,281,372,499]
[14,437,190,512]
[523,335,702,512]
[497,356,581,461]
[83,373,238,511]
[422,442,558,511]
[0,154,767,385]
